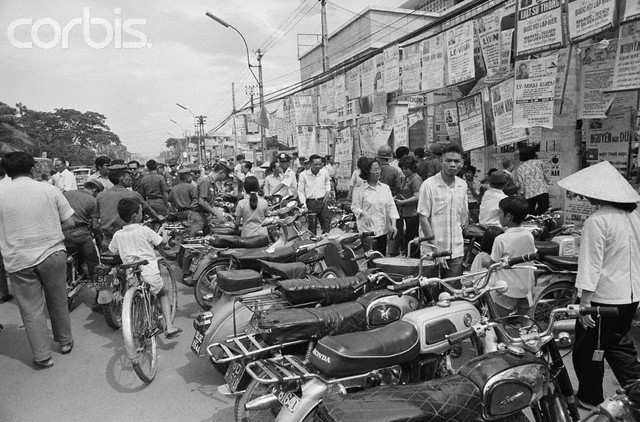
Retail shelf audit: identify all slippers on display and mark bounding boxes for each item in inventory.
[165,327,183,338]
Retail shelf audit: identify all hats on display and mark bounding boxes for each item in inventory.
[276,152,292,162]
[84,179,104,191]
[178,168,194,175]
[556,160,640,203]
[487,170,510,184]
[169,159,177,166]
[107,164,134,174]
[217,161,234,178]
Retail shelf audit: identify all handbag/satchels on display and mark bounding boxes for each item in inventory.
[388,196,404,240]
[395,194,406,206]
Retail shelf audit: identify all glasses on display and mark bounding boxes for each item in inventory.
[369,167,382,173]
[128,168,137,171]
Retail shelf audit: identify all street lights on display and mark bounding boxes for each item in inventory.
[169,118,190,164]
[175,102,202,166]
[204,10,267,161]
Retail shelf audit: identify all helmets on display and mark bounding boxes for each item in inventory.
[375,145,394,159]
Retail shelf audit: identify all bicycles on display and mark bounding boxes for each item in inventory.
[121,254,178,383]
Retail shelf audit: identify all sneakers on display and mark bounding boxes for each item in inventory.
[90,304,105,314]
[0,295,13,304]
[182,276,195,287]
[572,394,597,409]
[60,340,74,354]
[33,358,54,367]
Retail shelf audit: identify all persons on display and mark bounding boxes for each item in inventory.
[293,152,340,178]
[103,197,182,337]
[515,145,551,215]
[478,194,536,323]
[167,169,199,220]
[471,227,503,278]
[140,160,172,224]
[418,143,469,288]
[264,160,284,233]
[1,156,14,305]
[1,153,75,367]
[48,159,77,193]
[92,165,166,314]
[390,140,444,181]
[204,154,272,188]
[557,160,638,412]
[377,145,397,189]
[86,155,201,188]
[269,153,298,215]
[232,177,270,238]
[62,180,102,280]
[351,159,400,269]
[463,145,522,227]
[298,156,331,239]
[393,157,424,258]
[346,157,368,201]
[180,160,230,286]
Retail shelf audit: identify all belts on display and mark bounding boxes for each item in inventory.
[146,196,159,199]
[178,207,188,211]
[191,208,207,214]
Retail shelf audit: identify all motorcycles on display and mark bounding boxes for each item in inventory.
[153,189,364,262]
[453,198,582,254]
[532,254,588,348]
[94,214,165,332]
[192,231,379,378]
[316,304,619,421]
[250,254,542,422]
[205,251,452,421]
[232,235,437,388]
[66,227,102,308]
